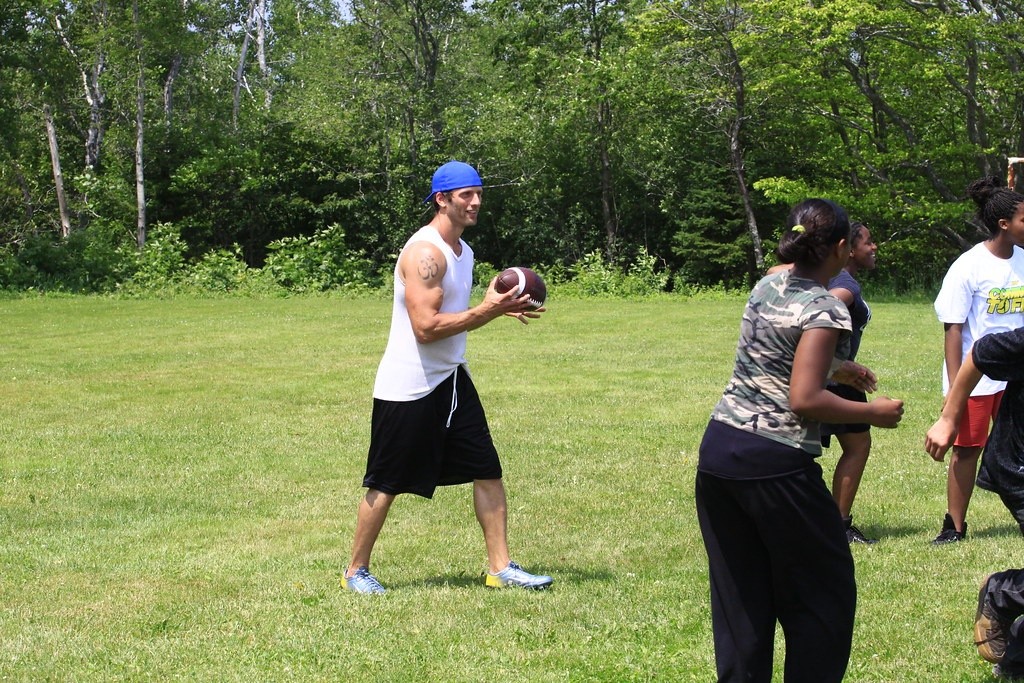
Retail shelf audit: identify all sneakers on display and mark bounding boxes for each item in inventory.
[931,513,967,545]
[486,561,553,593]
[974,574,1009,663]
[341,566,388,596]
[842,514,880,545]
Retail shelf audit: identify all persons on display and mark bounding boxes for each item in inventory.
[932,174,1024,544]
[926,326,1024,682]
[767,221,878,544]
[339,161,553,594]
[694,199,905,683]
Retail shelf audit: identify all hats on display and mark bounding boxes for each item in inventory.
[423,161,483,204]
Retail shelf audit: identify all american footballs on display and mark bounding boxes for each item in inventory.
[497,267,546,311]
